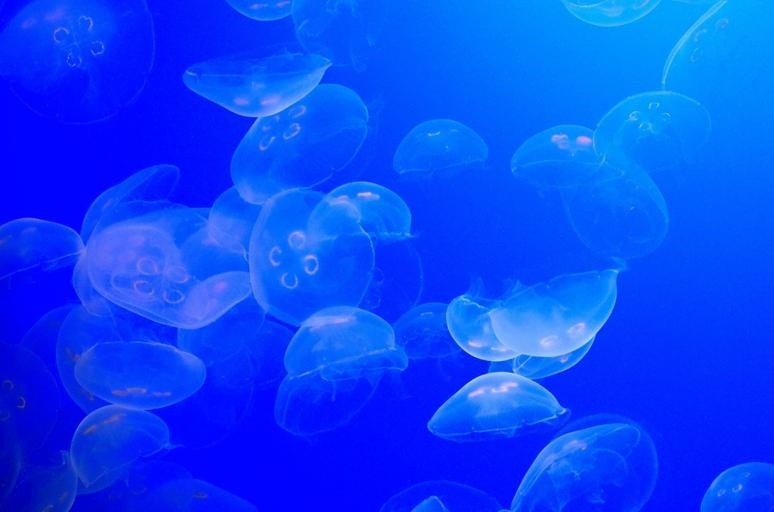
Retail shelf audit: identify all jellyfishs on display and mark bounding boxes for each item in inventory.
[0,1,774,511]
